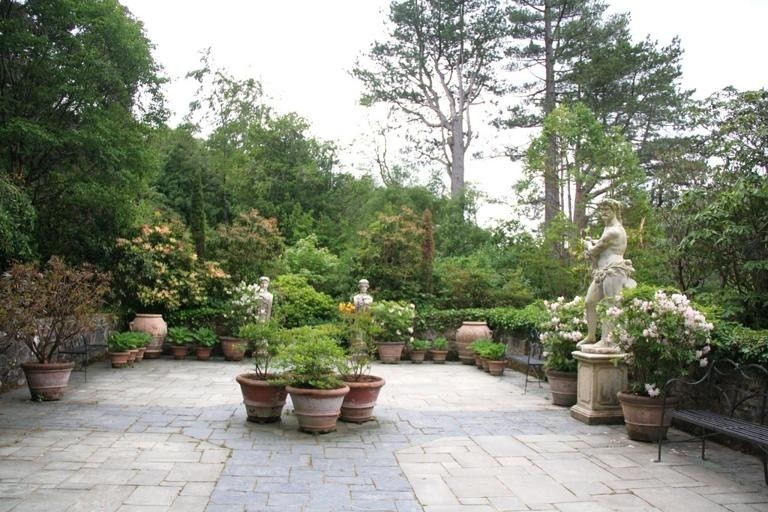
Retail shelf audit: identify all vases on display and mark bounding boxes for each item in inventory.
[220,336,249,361]
[616,391,680,441]
[378,341,405,364]
[545,367,578,406]
[21,362,76,401]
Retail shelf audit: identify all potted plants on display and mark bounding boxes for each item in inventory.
[167,327,194,359]
[195,325,218,359]
[235,320,384,434]
[430,336,449,362]
[466,339,512,375]
[409,338,430,362]
[108,330,153,368]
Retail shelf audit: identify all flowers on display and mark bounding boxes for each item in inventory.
[368,298,418,342]
[603,290,714,397]
[538,295,588,372]
[224,281,266,337]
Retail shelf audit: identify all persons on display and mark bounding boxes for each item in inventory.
[575,197,634,349]
[353,278,374,353]
[254,275,274,352]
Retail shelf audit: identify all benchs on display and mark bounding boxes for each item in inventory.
[652,357,768,485]
